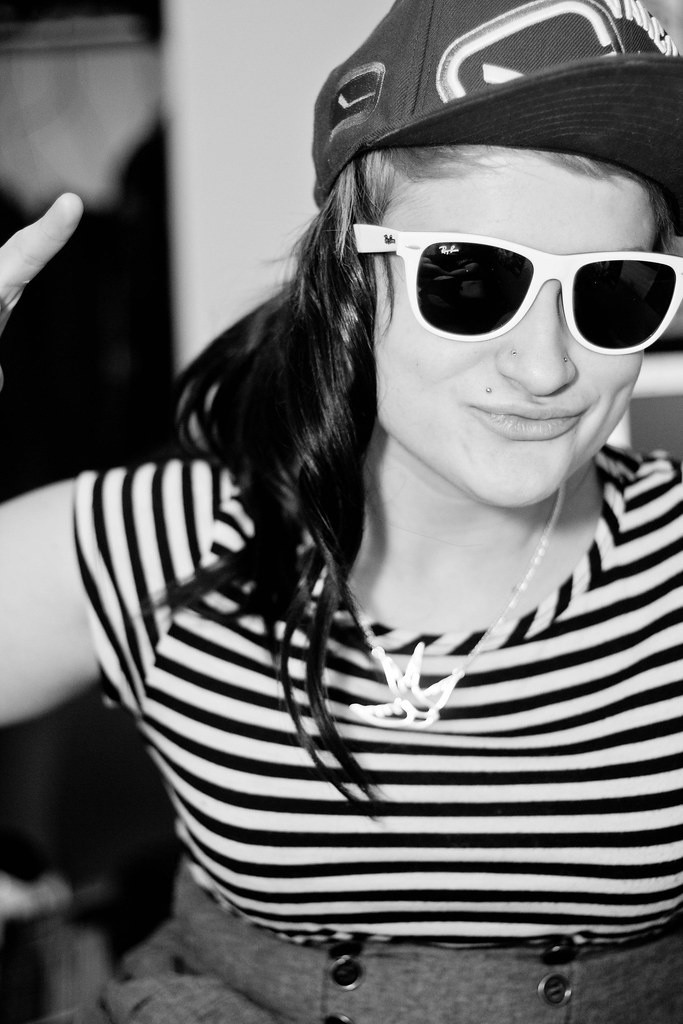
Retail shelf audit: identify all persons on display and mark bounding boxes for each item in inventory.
[1,0,683,1024]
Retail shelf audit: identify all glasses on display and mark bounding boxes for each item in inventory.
[354,223,683,355]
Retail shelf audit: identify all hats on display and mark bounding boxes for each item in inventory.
[312,0,683,207]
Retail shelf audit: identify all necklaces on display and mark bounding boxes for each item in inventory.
[351,484,565,728]
